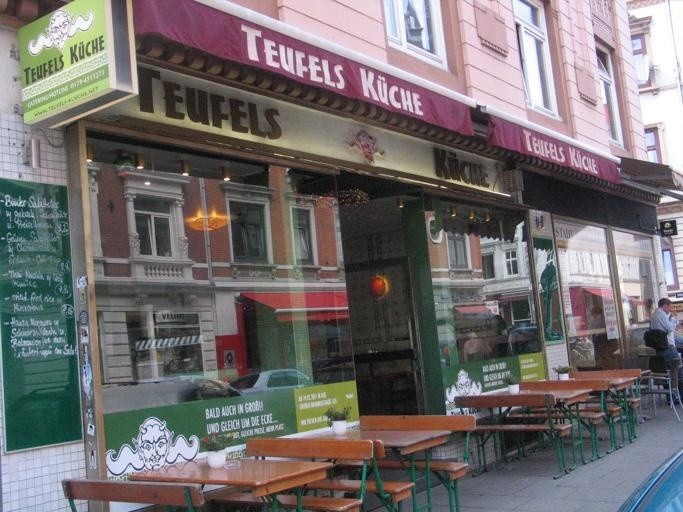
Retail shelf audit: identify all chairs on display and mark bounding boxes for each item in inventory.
[641,357,683,423]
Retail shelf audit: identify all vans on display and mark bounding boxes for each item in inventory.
[306,345,425,417]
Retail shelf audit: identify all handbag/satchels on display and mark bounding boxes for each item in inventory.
[644,329,668,350]
[592,333,619,355]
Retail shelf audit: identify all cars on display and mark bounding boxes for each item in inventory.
[226,369,311,399]
[435,311,509,367]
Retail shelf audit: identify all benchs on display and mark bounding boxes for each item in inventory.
[61,415,474,512]
[452,369,642,481]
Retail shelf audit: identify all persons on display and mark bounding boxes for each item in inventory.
[627,318,639,330]
[650,298,681,406]
[584,306,618,395]
